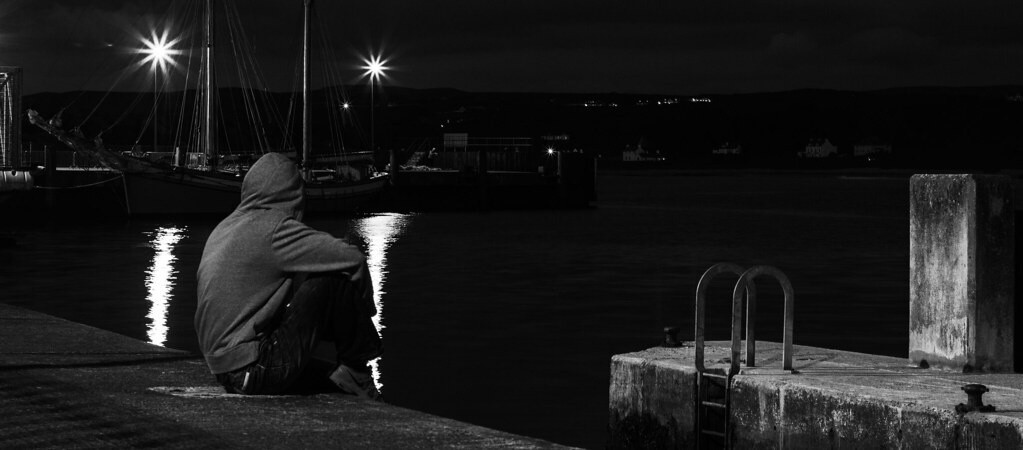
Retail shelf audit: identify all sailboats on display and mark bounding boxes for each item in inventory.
[25,0,388,220]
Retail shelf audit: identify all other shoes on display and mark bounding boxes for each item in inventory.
[330,365,382,402]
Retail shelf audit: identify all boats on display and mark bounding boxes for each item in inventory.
[621,138,673,170]
[390,131,598,212]
[797,138,837,160]
[712,142,741,157]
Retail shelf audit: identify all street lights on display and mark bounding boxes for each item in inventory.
[140,34,177,151]
[357,52,387,151]
[544,144,562,185]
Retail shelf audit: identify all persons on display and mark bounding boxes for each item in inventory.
[193,151,384,402]
